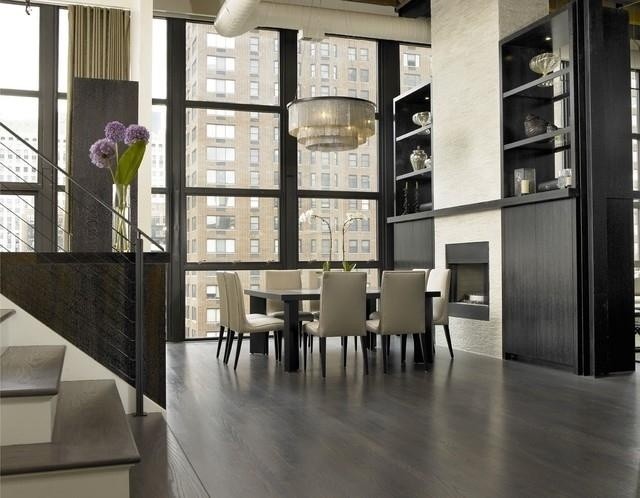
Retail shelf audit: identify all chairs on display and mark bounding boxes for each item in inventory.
[216,268,454,377]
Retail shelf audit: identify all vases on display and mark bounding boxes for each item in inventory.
[111,183,131,252]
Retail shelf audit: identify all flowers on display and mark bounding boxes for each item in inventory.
[88,120,149,250]
[299,208,368,272]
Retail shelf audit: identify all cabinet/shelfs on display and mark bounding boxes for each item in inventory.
[393,79,435,270]
[498,3,635,378]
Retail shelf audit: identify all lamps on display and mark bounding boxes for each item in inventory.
[286,1,377,150]
